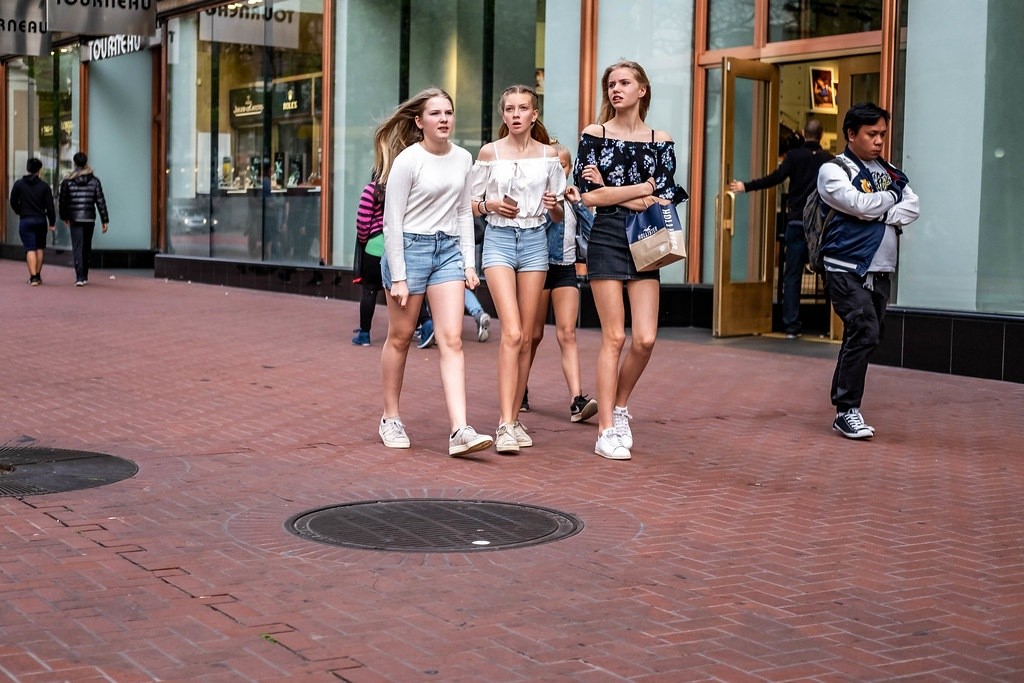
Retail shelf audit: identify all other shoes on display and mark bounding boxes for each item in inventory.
[786,331,803,338]
[28,277,42,286]
[75,280,87,286]
[477,313,490,342]
[412,323,422,339]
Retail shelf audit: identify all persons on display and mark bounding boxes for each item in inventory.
[730,118,835,339]
[353,173,490,349]
[817,101,920,438]
[472,85,567,452]
[59,153,109,286]
[573,61,689,459]
[519,140,599,423]
[10,158,56,286]
[246,164,318,260]
[373,89,492,456]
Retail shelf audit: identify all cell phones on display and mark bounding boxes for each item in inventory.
[503,193,518,208]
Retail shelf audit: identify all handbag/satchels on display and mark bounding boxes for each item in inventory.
[625,194,687,272]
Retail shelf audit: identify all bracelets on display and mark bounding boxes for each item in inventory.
[478,201,484,214]
[647,181,654,190]
[482,201,490,213]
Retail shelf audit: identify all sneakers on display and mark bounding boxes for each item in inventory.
[513,421,532,447]
[378,416,410,448]
[352,328,371,346]
[570,390,598,422]
[594,427,631,460]
[832,408,875,438]
[417,320,435,349]
[614,405,633,449]
[494,422,519,451]
[448,426,493,456]
[519,390,530,411]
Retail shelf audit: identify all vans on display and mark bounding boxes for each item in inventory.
[172,204,205,233]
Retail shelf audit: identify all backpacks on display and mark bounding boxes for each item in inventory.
[802,156,851,276]
